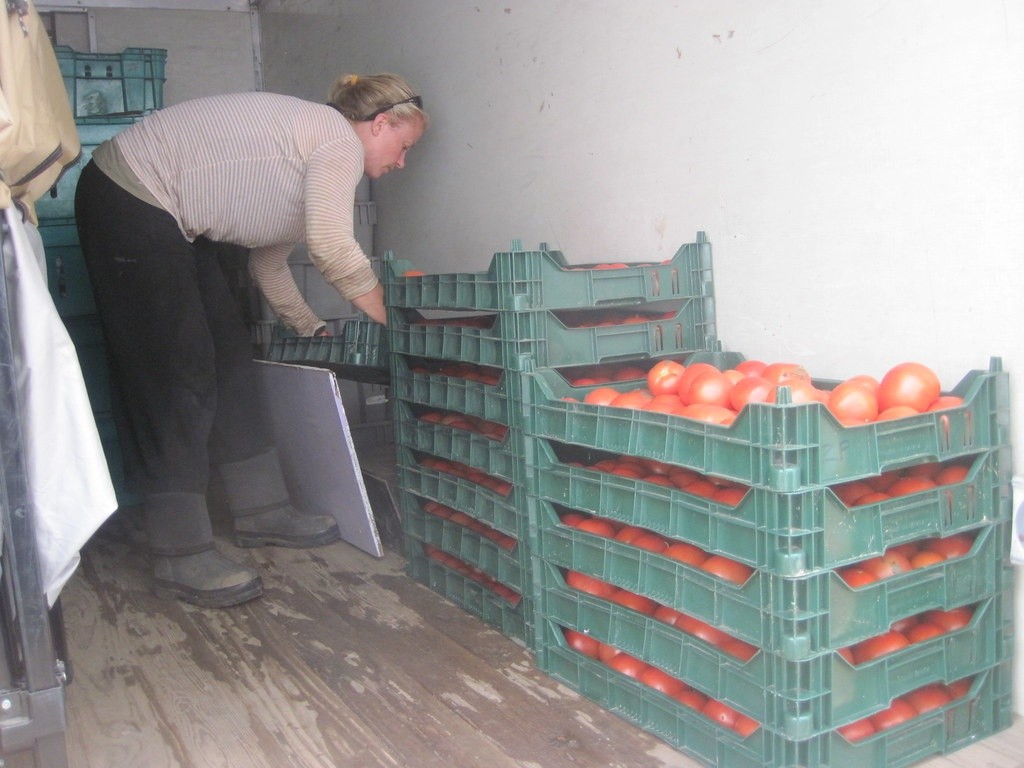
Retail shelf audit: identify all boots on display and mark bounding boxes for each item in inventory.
[142,491,265,607]
[216,445,341,549]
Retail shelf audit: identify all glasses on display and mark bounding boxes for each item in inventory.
[362,94,423,122]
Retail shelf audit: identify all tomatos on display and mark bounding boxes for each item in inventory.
[401,259,983,748]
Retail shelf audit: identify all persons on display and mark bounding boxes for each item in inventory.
[74,75,429,610]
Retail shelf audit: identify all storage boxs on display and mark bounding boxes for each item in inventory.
[31,39,1024,768]
